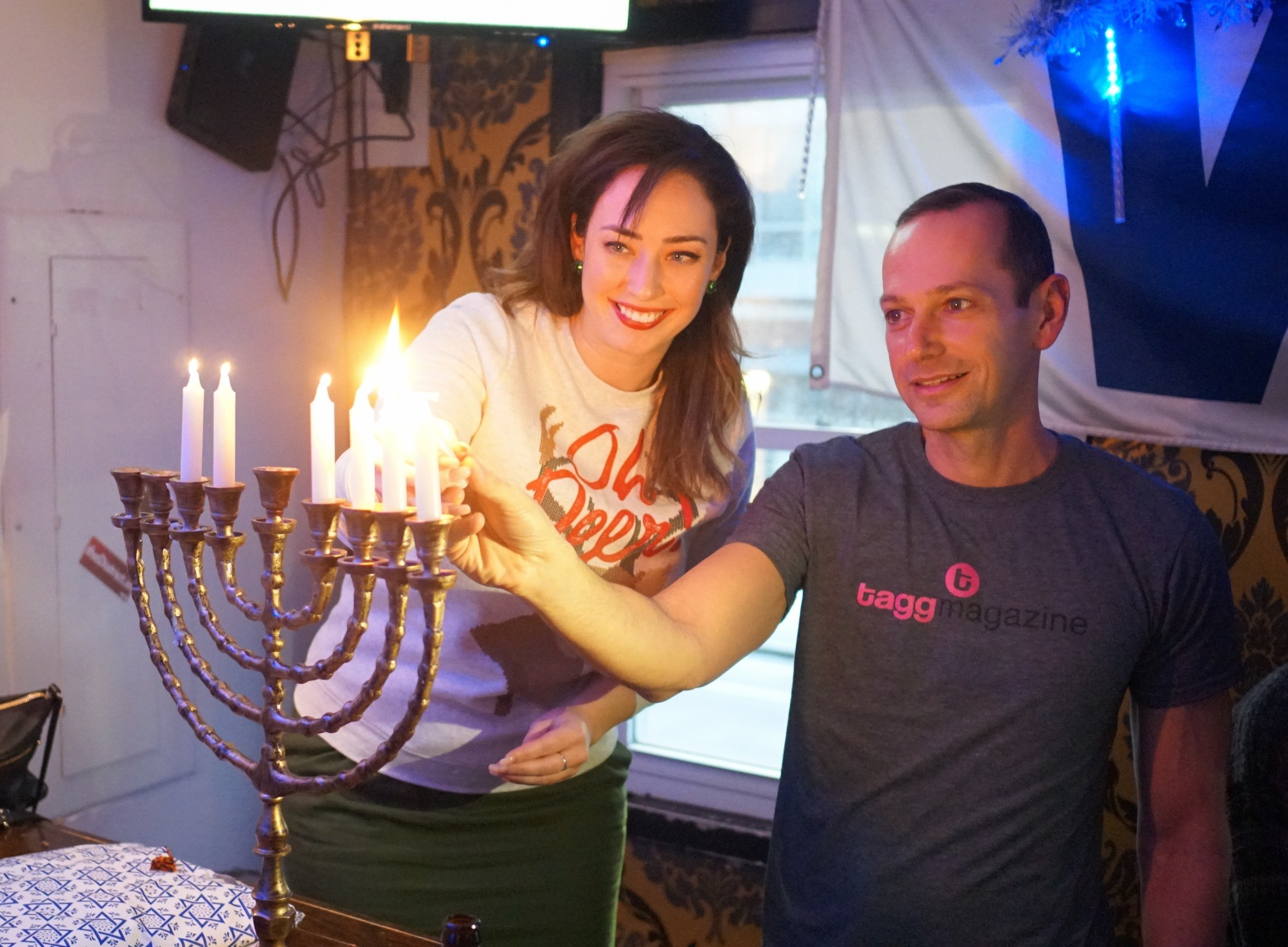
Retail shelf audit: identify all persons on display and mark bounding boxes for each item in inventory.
[256,109,757,947]
[441,181,1244,947]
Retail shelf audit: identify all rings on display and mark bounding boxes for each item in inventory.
[558,751,567,770]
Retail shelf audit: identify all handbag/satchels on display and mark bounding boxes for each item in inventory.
[0,684,62,831]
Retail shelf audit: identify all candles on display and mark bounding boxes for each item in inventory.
[174,356,445,521]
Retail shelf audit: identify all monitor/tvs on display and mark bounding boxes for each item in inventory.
[143,0,641,50]
